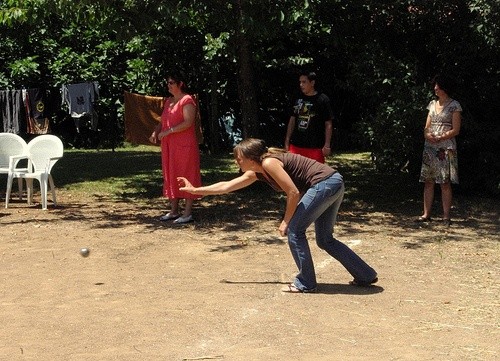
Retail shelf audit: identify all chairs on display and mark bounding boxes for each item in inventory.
[0,132,34,203]
[4,133,64,211]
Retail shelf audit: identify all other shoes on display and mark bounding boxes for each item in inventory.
[159,213,177,221]
[173,215,192,224]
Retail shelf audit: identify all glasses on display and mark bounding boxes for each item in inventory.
[167,81,175,84]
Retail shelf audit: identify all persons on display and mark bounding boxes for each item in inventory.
[150,68,202,223]
[412,75,463,222]
[176,138,378,293]
[284,68,334,163]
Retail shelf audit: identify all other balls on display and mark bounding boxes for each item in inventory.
[80,247,90,257]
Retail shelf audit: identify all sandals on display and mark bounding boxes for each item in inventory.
[281,284,303,293]
[351,276,378,285]
